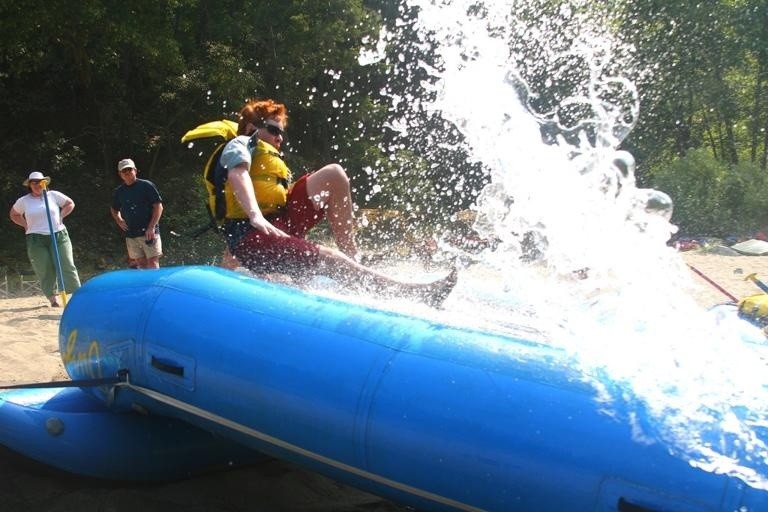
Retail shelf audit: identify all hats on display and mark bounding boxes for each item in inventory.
[23,170,51,188]
[117,158,136,172]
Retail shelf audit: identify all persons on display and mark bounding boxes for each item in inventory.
[7,171,81,306]
[178,99,458,310]
[108,158,167,271]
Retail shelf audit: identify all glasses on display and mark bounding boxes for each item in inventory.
[258,121,285,137]
